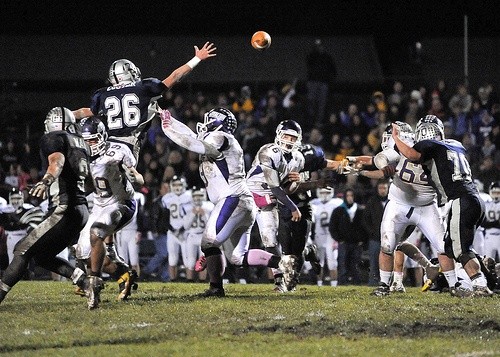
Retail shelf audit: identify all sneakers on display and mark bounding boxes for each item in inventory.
[306,244,322,274]
[194,255,207,272]
[371,256,500,299]
[275,278,297,291]
[278,255,300,291]
[74,271,106,311]
[198,283,225,298]
[115,260,139,303]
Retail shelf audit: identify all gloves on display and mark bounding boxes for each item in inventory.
[28,173,56,200]
[336,156,359,177]
[129,167,144,187]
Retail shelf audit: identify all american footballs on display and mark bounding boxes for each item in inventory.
[251,31,272,52]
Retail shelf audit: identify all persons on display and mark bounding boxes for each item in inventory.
[71,41,218,266]
[78,117,144,312]
[0,105,104,311]
[0,78,500,311]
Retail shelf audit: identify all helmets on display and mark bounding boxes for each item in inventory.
[109,59,142,85]
[474,178,500,203]
[195,106,237,137]
[380,114,445,152]
[316,185,335,202]
[44,105,76,134]
[80,117,108,158]
[169,175,187,196]
[191,186,207,206]
[9,187,24,207]
[275,119,303,155]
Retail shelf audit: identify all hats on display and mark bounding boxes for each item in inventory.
[373,91,384,100]
